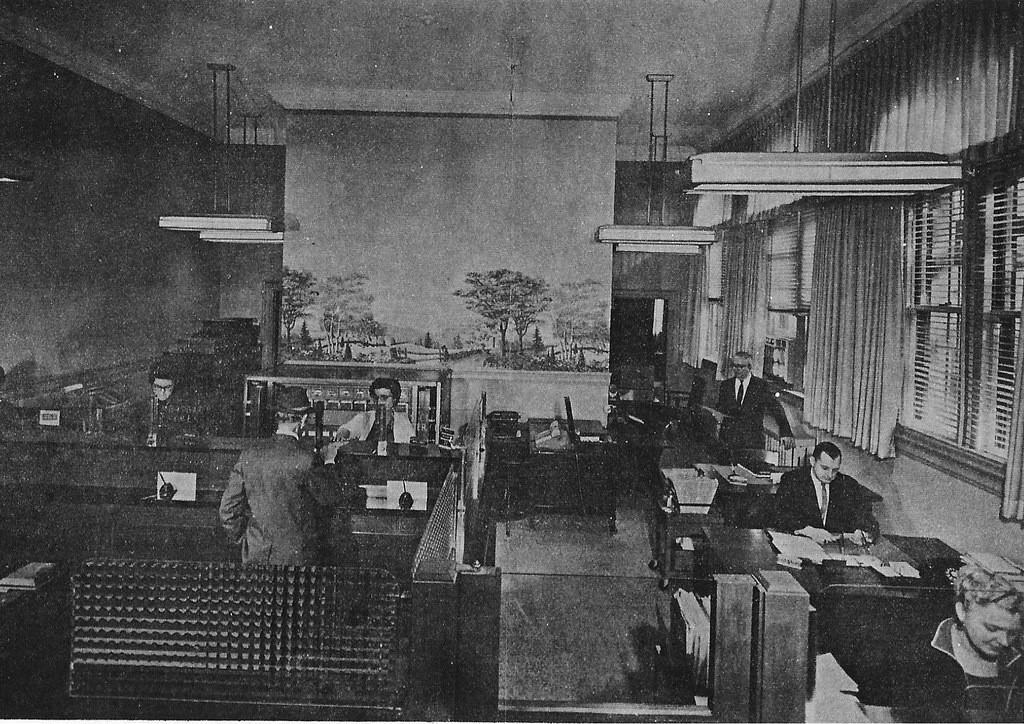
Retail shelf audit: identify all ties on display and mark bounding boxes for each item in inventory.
[736,380,743,413]
[820,482,827,526]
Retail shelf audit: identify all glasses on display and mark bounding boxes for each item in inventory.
[371,394,393,402]
[732,364,748,370]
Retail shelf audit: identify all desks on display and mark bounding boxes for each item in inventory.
[529,416,620,537]
[647,466,885,591]
[242,368,452,437]
[701,524,969,587]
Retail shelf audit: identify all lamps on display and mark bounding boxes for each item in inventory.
[156,213,284,243]
[598,219,720,255]
[678,151,962,197]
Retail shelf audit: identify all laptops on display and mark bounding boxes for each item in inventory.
[564,397,608,437]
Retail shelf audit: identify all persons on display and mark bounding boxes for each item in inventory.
[891,571,1024,723]
[130,362,202,435]
[712,352,795,467]
[219,387,338,564]
[0,368,21,461]
[331,378,416,444]
[772,443,879,544]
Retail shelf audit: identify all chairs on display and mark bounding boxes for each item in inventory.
[667,376,706,410]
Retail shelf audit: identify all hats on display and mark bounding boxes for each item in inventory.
[275,386,311,411]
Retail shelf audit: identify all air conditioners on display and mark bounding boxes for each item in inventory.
[763,334,797,387]
